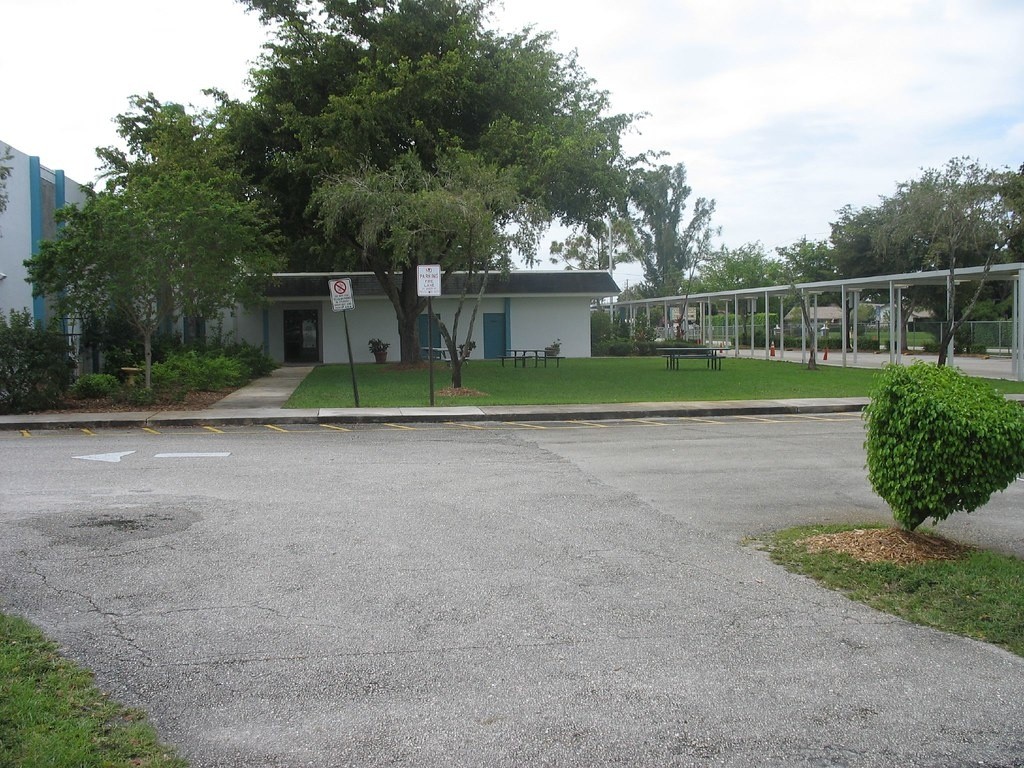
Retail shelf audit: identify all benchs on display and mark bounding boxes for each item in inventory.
[661,354,718,370]
[533,356,565,367]
[671,356,726,370]
[442,357,470,367]
[420,355,440,362]
[497,355,532,367]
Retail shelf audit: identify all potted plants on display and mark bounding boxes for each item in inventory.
[457,341,476,357]
[368,338,390,363]
[545,338,562,356]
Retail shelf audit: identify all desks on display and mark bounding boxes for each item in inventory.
[656,347,731,370]
[420,347,460,360]
[505,349,556,368]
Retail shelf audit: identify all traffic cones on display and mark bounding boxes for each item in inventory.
[769,340,776,356]
